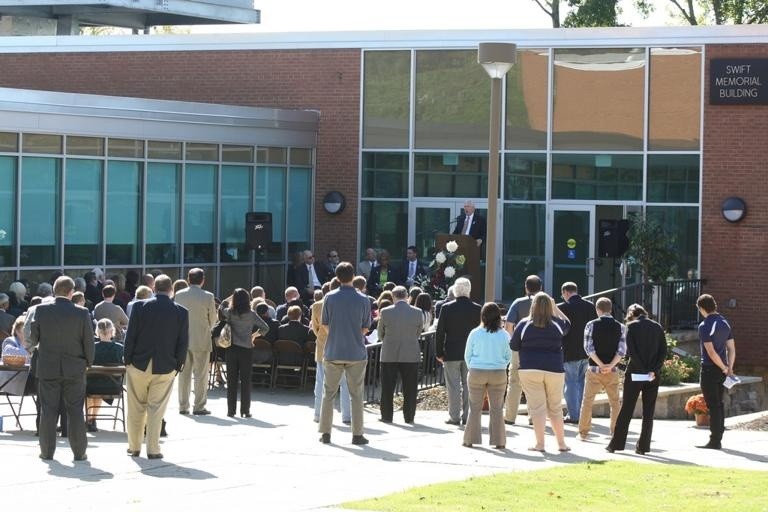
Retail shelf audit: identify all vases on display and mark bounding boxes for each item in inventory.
[695,414,710,428]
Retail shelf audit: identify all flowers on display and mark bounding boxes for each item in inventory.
[684,393,710,415]
[407,240,466,299]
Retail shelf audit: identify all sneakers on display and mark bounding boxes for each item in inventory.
[575,434,586,441]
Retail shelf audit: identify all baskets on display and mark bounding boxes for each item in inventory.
[2,354,26,368]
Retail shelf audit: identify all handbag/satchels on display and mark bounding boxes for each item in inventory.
[214,309,231,348]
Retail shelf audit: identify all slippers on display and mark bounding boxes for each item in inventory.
[527,447,545,452]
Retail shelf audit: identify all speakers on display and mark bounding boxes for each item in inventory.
[598,219,629,257]
[246,212,272,249]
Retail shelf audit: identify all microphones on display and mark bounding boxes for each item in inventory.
[456,215,466,223]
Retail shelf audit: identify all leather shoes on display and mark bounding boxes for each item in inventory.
[179,410,189,414]
[694,441,720,449]
[319,434,330,443]
[352,436,368,445]
[193,409,211,415]
[126,449,139,456]
[147,454,163,459]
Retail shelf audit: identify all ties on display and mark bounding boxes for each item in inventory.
[409,263,414,276]
[309,266,313,290]
[461,216,470,235]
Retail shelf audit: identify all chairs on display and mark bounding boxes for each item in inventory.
[0,328,40,431]
[209,338,315,393]
[85,364,125,432]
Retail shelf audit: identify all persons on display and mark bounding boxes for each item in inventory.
[356,248,374,283]
[0,265,600,367]
[391,247,427,285]
[508,292,571,454]
[223,287,268,418]
[462,303,511,450]
[435,277,482,425]
[317,261,371,444]
[326,250,340,275]
[378,285,424,424]
[83,316,125,431]
[694,295,735,449]
[368,251,400,295]
[299,250,324,291]
[29,276,95,461]
[606,303,666,453]
[312,277,353,423]
[556,281,599,422]
[574,297,627,442]
[2,318,68,436]
[176,268,217,414]
[121,274,189,459]
[127,286,168,436]
[451,200,484,246]
[504,274,542,424]
